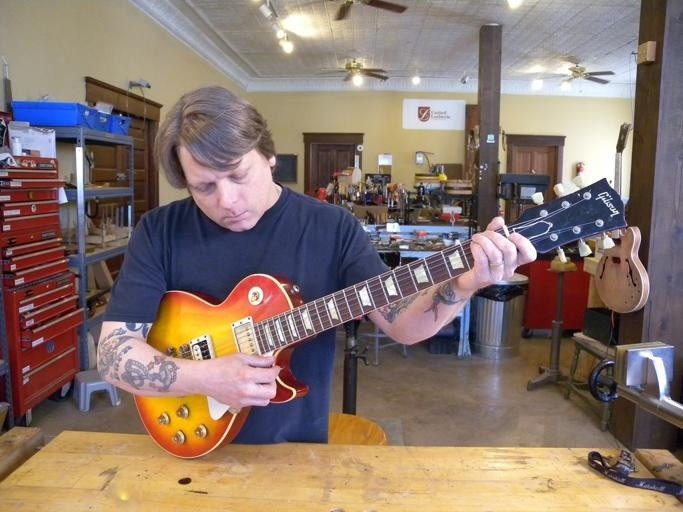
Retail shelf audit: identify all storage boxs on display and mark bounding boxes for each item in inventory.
[12,101,133,136]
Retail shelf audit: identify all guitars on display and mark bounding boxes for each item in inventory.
[132,179,627,458]
[595,122,650,314]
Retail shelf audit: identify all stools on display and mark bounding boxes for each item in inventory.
[327,411,386,447]
[563,331,617,434]
[73,369,122,411]
[356,319,407,365]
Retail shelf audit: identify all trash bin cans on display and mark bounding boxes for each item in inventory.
[468,272,529,360]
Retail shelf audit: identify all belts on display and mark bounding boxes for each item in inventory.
[588,449,683,496]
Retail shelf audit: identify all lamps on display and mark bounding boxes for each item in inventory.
[279,38,292,54]
[270,22,284,39]
[259,0,272,19]
[461,76,468,84]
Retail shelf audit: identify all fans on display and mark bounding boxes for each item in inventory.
[322,0,407,20]
[538,61,614,84]
[322,60,389,82]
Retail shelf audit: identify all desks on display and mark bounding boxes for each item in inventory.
[0,429,683,511]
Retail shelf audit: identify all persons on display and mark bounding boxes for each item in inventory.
[96,86,537,445]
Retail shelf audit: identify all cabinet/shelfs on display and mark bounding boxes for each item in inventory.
[52,125,135,369]
